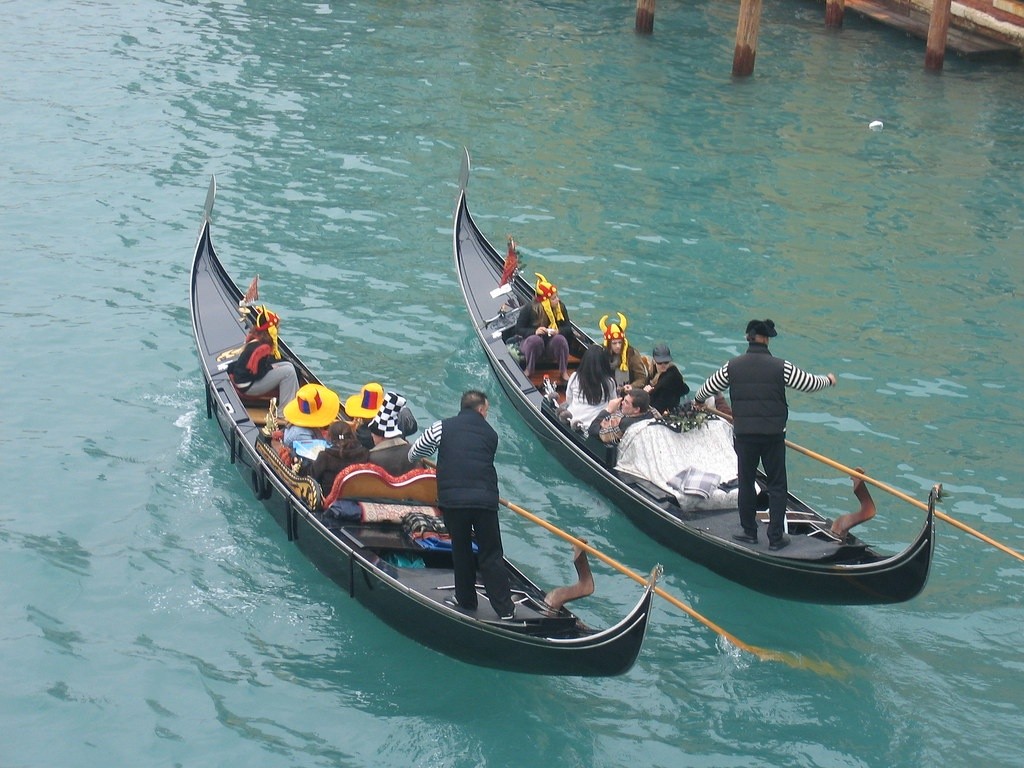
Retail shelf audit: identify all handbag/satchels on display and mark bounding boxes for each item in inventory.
[402,407,418,437]
[401,512,449,541]
[681,383,690,396]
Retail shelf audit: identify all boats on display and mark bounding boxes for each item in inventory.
[186,171,665,681]
[450,145,944,610]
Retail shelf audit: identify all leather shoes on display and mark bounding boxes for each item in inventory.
[769,537,790,551]
[732,531,758,544]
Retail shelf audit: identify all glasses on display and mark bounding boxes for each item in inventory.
[654,359,669,364]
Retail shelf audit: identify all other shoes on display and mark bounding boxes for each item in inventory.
[524,369,530,378]
[561,370,570,382]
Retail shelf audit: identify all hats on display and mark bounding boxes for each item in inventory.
[256,304,281,360]
[745,319,777,337]
[344,382,384,418]
[600,312,629,372]
[653,344,673,363]
[284,383,339,427]
[368,391,407,439]
[534,273,565,331]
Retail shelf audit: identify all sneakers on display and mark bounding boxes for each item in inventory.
[445,594,479,610]
[502,606,516,620]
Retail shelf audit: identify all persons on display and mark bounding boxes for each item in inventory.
[517,272,571,382]
[407,390,516,619]
[694,319,836,550]
[232,304,422,496]
[566,312,684,447]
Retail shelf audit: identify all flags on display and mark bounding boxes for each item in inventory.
[497,243,521,287]
[241,274,259,301]
